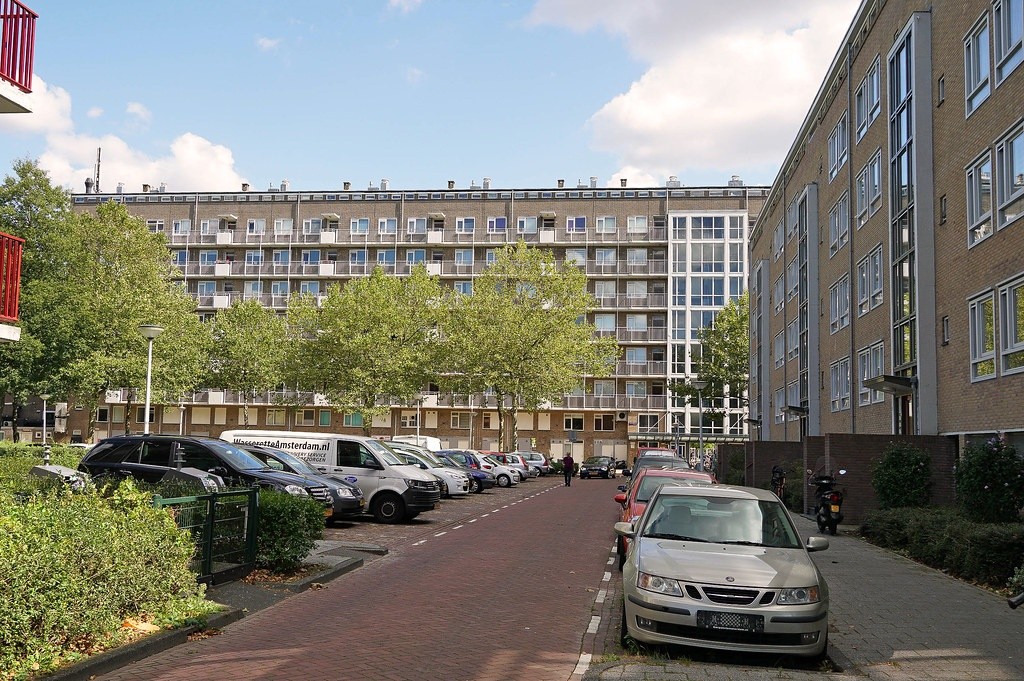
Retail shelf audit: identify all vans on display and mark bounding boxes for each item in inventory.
[219,429,442,524]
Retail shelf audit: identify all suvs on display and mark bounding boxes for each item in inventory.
[77,436,337,524]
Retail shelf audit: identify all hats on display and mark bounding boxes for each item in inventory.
[567,452,570,455]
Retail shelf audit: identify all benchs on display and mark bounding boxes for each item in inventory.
[692,514,724,540]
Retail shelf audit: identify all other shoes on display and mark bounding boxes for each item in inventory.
[568,483,570,487]
[565,483,568,486]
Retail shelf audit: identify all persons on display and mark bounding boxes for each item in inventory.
[563,452,574,487]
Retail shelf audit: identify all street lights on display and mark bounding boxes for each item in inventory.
[177,406,187,435]
[137,324,164,435]
[412,393,423,447]
[40,394,50,445]
[692,381,709,473]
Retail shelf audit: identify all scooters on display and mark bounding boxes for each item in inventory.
[806,455,846,536]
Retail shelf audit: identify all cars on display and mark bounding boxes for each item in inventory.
[614,447,830,669]
[382,440,549,500]
[237,444,365,518]
[579,455,616,478]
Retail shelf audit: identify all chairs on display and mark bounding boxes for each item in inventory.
[655,505,696,537]
